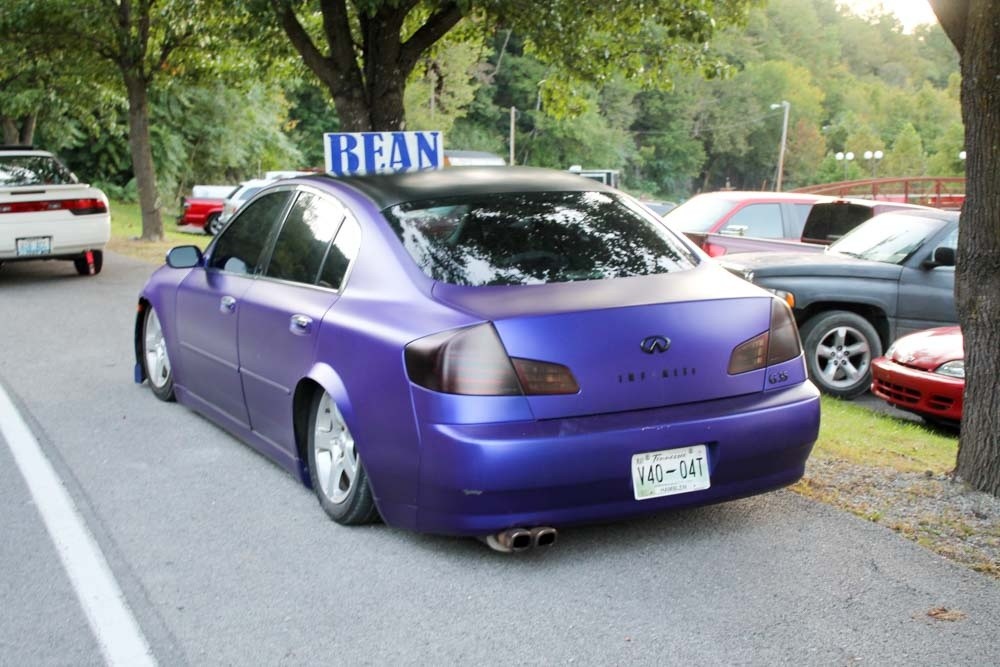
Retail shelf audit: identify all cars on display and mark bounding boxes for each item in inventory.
[0,143,112,280]
[867,325,966,432]
[131,129,824,559]
[174,149,679,239]
[712,208,964,402]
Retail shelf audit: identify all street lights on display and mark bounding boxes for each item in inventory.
[835,151,855,181]
[863,150,883,179]
[770,100,791,192]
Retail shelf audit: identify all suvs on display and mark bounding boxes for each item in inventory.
[660,189,960,260]
